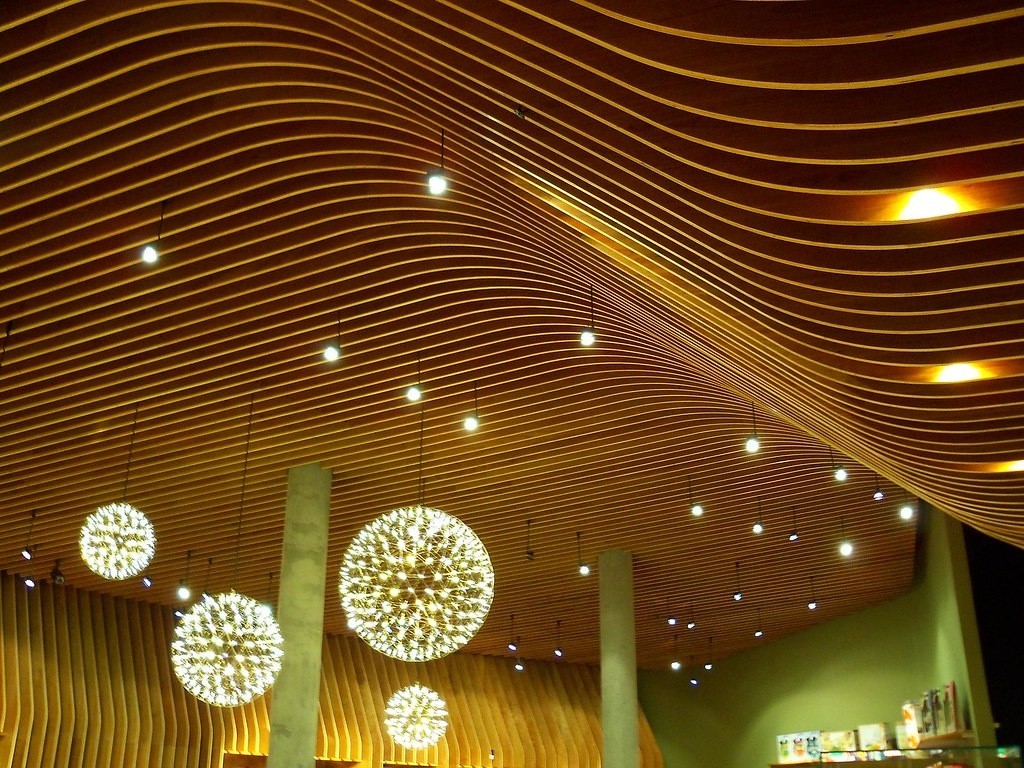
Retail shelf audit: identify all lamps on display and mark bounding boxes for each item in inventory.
[21,127,883,755]
